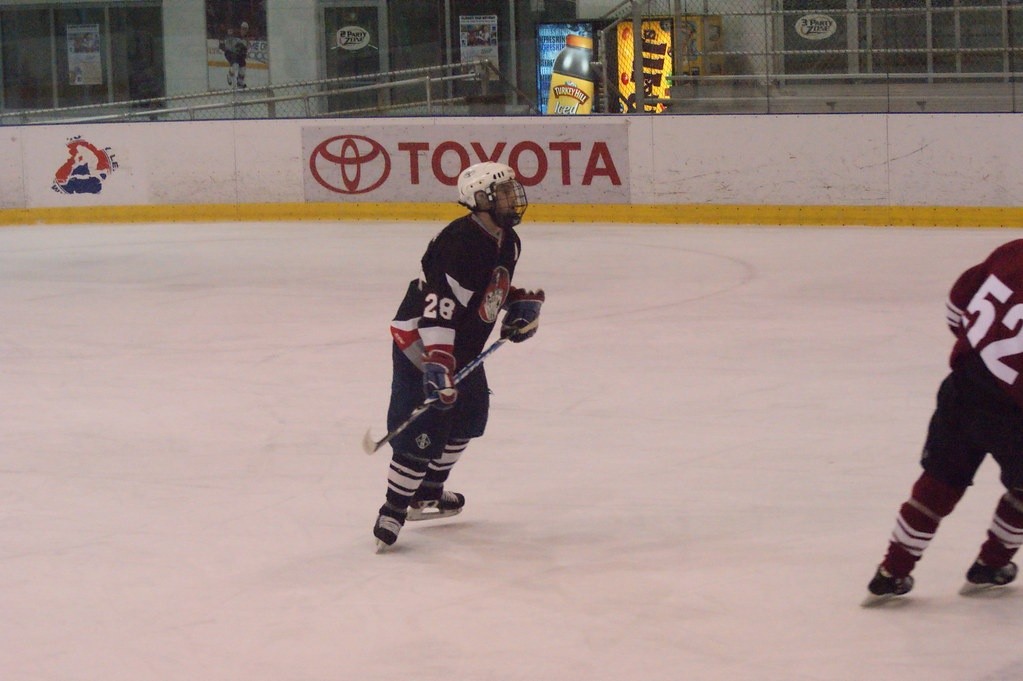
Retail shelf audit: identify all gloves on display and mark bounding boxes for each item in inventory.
[421,350,458,417]
[500,288,544,343]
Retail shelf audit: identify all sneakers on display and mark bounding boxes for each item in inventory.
[959,560,1017,593]
[406,491,465,521]
[860,568,914,606]
[374,503,408,553]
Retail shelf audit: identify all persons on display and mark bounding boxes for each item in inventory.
[863,238,1023,609]
[467,25,489,46]
[372,162,544,553]
[218,20,248,89]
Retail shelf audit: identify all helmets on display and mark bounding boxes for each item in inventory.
[457,161,528,227]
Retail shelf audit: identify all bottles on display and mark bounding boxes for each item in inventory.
[547,34,594,114]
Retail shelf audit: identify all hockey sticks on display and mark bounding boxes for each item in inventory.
[362,338,509,455]
